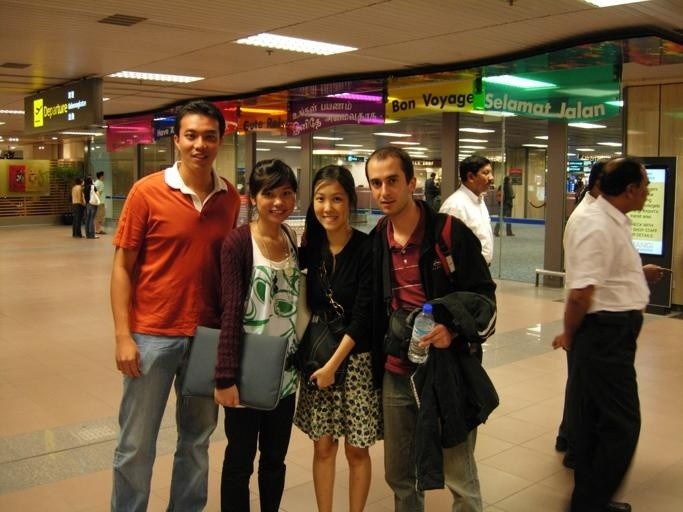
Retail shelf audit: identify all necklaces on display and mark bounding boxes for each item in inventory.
[252,219,292,315]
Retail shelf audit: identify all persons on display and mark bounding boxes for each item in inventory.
[574,173,585,206]
[365,146,497,511]
[554,156,604,466]
[71,171,107,239]
[214,158,303,512]
[109,101,241,512]
[424,156,516,265]
[567,159,677,511]
[295,164,385,512]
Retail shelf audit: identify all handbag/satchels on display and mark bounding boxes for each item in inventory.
[294,312,348,387]
[384,308,411,360]
[89,185,99,206]
[496,190,505,203]
[181,325,289,412]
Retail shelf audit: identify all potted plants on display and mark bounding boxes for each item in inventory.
[56,166,84,225]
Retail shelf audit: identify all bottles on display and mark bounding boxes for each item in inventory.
[407,304,435,364]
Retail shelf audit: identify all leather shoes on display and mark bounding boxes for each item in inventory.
[572,500,630,512]
